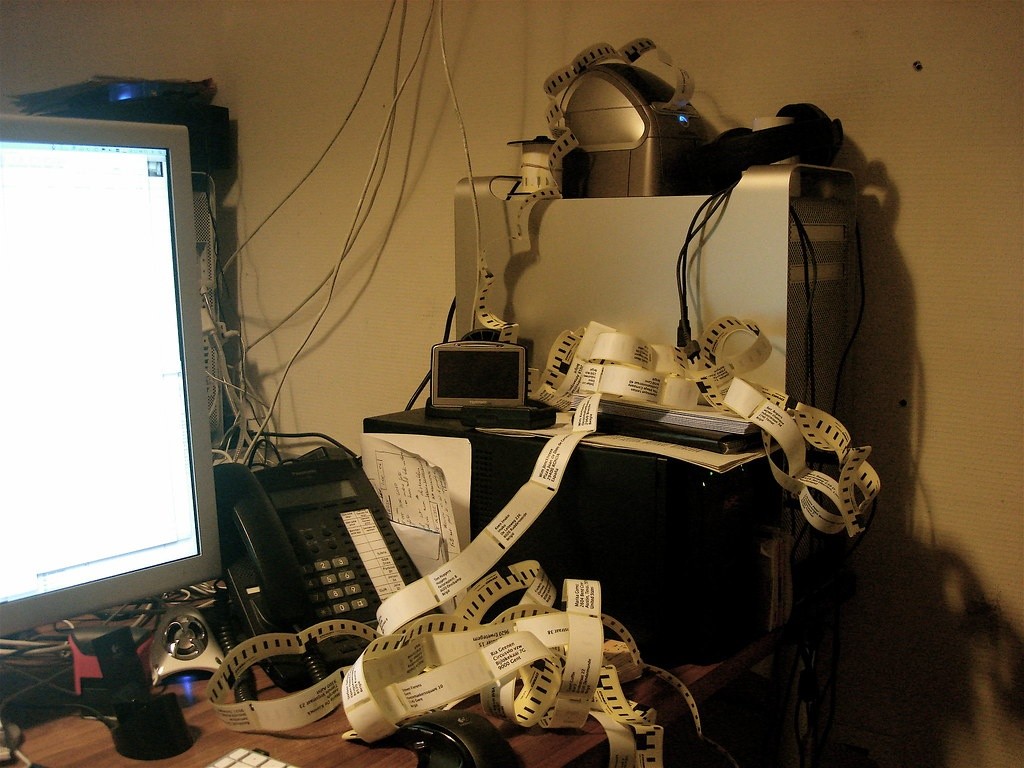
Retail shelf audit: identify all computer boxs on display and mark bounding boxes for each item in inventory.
[457,163,857,566]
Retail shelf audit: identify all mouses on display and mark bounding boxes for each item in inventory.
[397,709,524,768]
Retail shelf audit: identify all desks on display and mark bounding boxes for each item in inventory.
[0,580,771,768]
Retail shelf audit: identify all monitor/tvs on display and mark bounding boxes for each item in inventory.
[0,110,223,638]
[430,340,525,407]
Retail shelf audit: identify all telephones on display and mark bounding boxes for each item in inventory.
[211,444,445,697]
